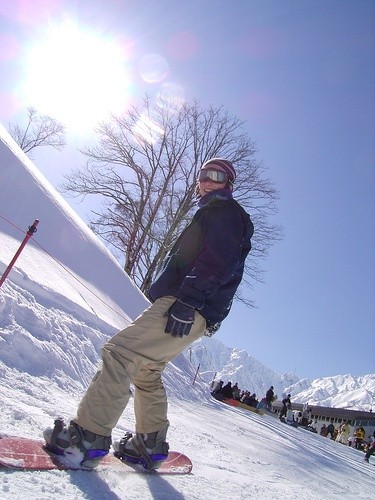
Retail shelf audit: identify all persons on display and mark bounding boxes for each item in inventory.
[354,425,365,449]
[212,380,257,408]
[310,420,316,429]
[336,421,350,445]
[320,424,326,435]
[279,394,292,417]
[266,385,273,411]
[43,159,256,469]
[326,420,335,438]
[363,431,375,463]
[281,405,295,422]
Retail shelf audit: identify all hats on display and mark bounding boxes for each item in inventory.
[201,158,237,185]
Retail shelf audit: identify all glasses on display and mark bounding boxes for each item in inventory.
[197,169,229,184]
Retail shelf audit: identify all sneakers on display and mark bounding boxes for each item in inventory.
[112,432,161,458]
[42,425,105,451]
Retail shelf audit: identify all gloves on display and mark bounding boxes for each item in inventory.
[204,319,221,338]
[163,296,196,339]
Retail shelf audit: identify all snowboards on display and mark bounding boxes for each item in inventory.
[0,433,192,475]
